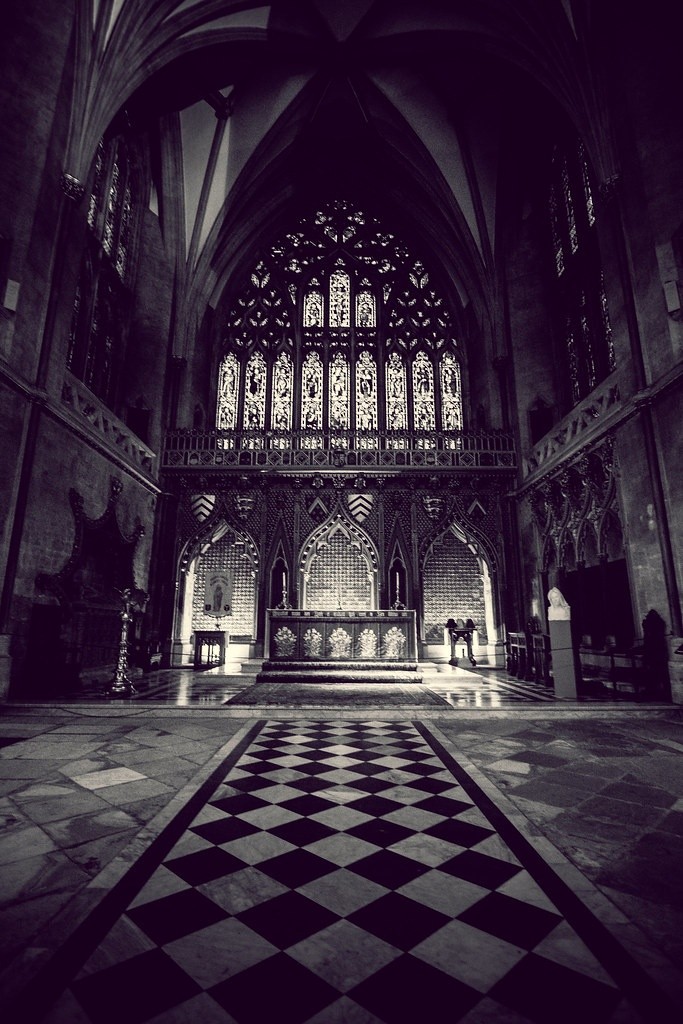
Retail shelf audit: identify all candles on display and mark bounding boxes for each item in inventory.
[282,572,286,589]
[396,572,399,589]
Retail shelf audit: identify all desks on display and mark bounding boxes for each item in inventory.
[193,630,228,671]
[264,609,418,663]
[449,627,477,666]
